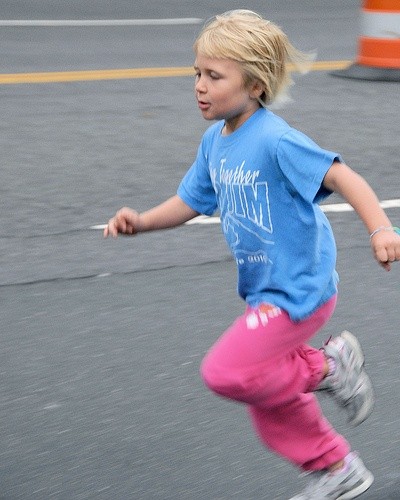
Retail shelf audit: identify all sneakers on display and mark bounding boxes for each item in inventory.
[289,450,374,500]
[318,330,373,429]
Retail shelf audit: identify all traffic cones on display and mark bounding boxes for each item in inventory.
[328,0,400,82]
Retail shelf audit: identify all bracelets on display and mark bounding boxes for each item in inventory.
[369,226,399,238]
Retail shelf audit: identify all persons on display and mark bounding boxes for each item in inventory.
[103,10,400,500]
